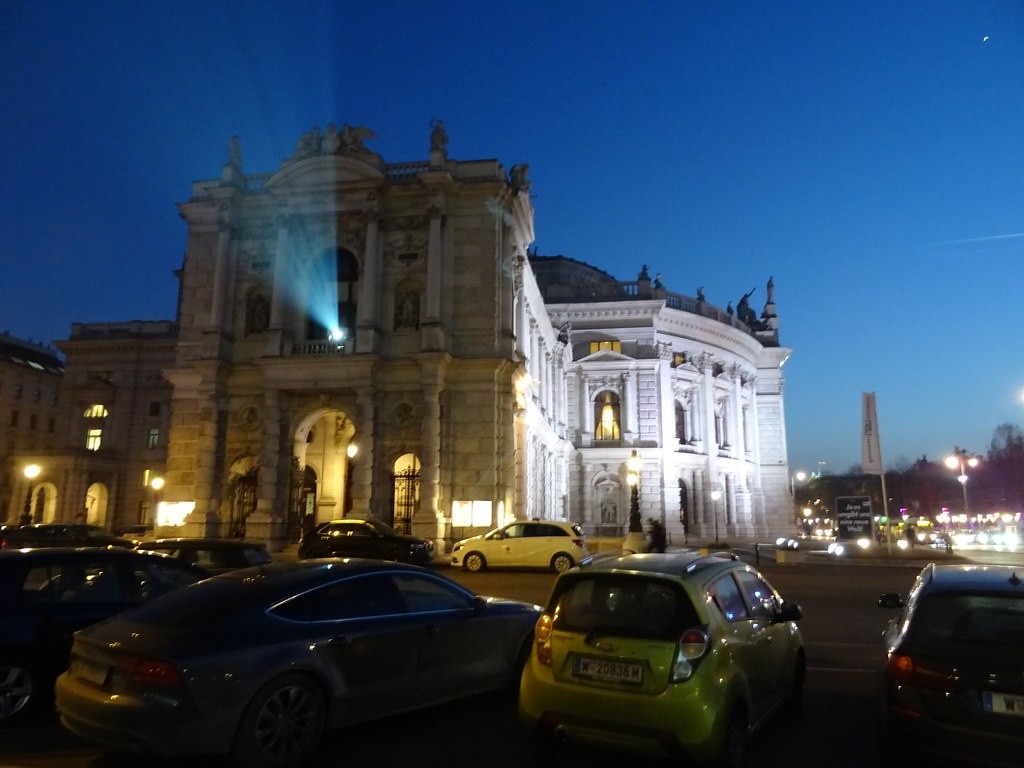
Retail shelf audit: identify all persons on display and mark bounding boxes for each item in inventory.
[429,117,447,150]
[875,528,881,545]
[227,136,241,167]
[646,518,666,553]
[602,500,615,523]
[249,294,268,332]
[509,164,532,192]
[321,124,340,154]
[906,526,915,547]
[727,301,733,312]
[654,273,663,288]
[767,276,774,303]
[697,287,704,298]
[642,265,648,280]
[401,287,418,325]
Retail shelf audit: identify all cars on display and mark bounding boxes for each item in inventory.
[450,518,588,573]
[0,547,215,745]
[140,538,274,574]
[516,550,807,768]
[55,556,548,768]
[876,563,1024,756]
[0,521,139,550]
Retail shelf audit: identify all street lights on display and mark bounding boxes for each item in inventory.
[944,454,979,527]
[18,463,40,529]
[792,470,805,508]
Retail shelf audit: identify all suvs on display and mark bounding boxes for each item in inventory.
[297,518,435,566]
